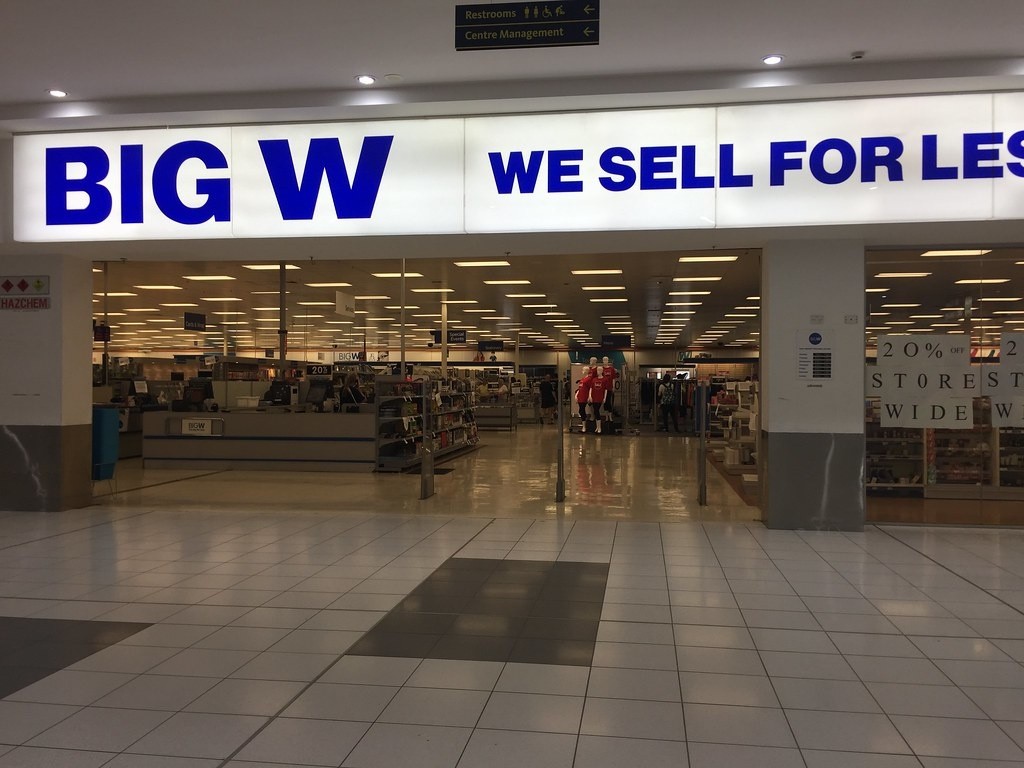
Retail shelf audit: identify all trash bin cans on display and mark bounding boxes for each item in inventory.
[92,402,122,480]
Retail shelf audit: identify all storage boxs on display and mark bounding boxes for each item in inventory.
[441,429,467,449]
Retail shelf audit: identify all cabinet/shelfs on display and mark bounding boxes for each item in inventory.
[866,399,926,488]
[374,374,480,469]
[926,409,1024,500]
